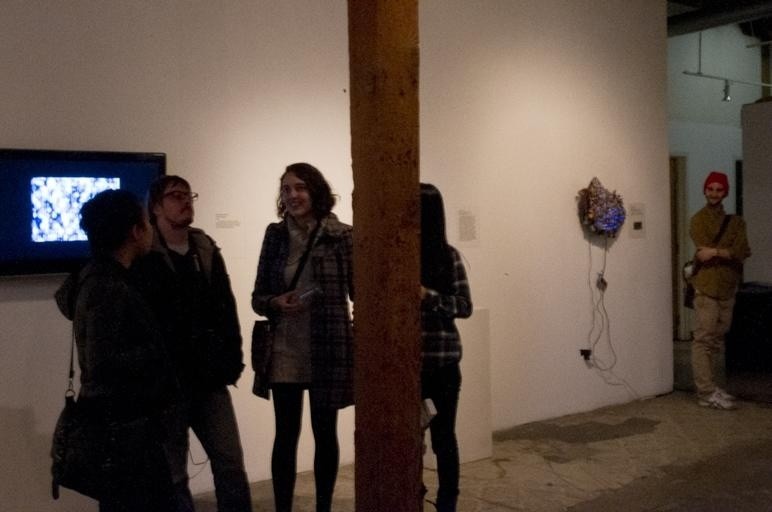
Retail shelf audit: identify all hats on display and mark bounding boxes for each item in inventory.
[703,170,730,198]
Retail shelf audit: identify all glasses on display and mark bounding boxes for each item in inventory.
[159,190,199,203]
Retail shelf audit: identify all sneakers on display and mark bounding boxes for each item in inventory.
[714,386,733,400]
[697,392,738,411]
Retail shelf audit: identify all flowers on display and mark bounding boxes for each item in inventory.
[578,175,625,238]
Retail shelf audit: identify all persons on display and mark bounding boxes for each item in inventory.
[251,163,357,510]
[684,170,752,409]
[54,191,190,511]
[418,182,473,511]
[143,175,250,512]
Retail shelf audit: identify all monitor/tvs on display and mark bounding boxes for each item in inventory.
[0,148,166,280]
